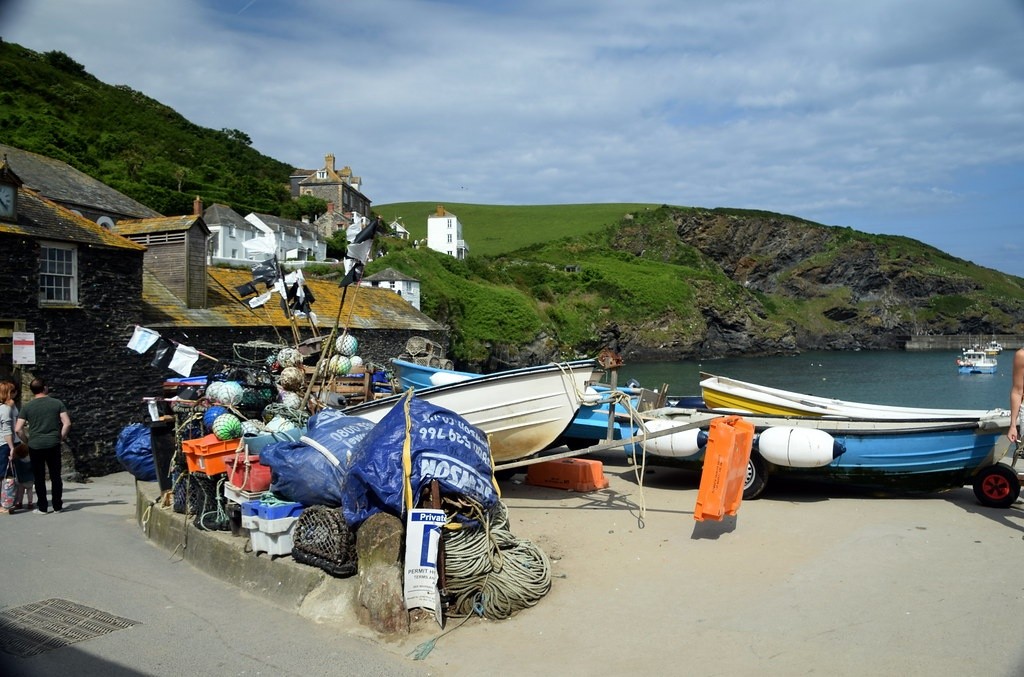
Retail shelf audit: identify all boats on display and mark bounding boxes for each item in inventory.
[701,376,1013,417]
[984,340,1002,354]
[958,349,999,374]
[342,359,595,463]
[622,410,1021,476]
[392,359,646,439]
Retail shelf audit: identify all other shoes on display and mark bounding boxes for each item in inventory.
[15,503,22,509]
[54,508,65,514]
[0,507,9,513]
[32,508,48,515]
[28,502,33,509]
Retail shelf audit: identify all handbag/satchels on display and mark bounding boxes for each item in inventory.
[0,457,19,508]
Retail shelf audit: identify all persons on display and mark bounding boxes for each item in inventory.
[15,377,71,515]
[1007,348,1024,442]
[0,380,19,514]
[15,444,35,509]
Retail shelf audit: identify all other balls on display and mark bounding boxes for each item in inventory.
[276,348,303,410]
[203,380,243,440]
[329,334,363,375]
